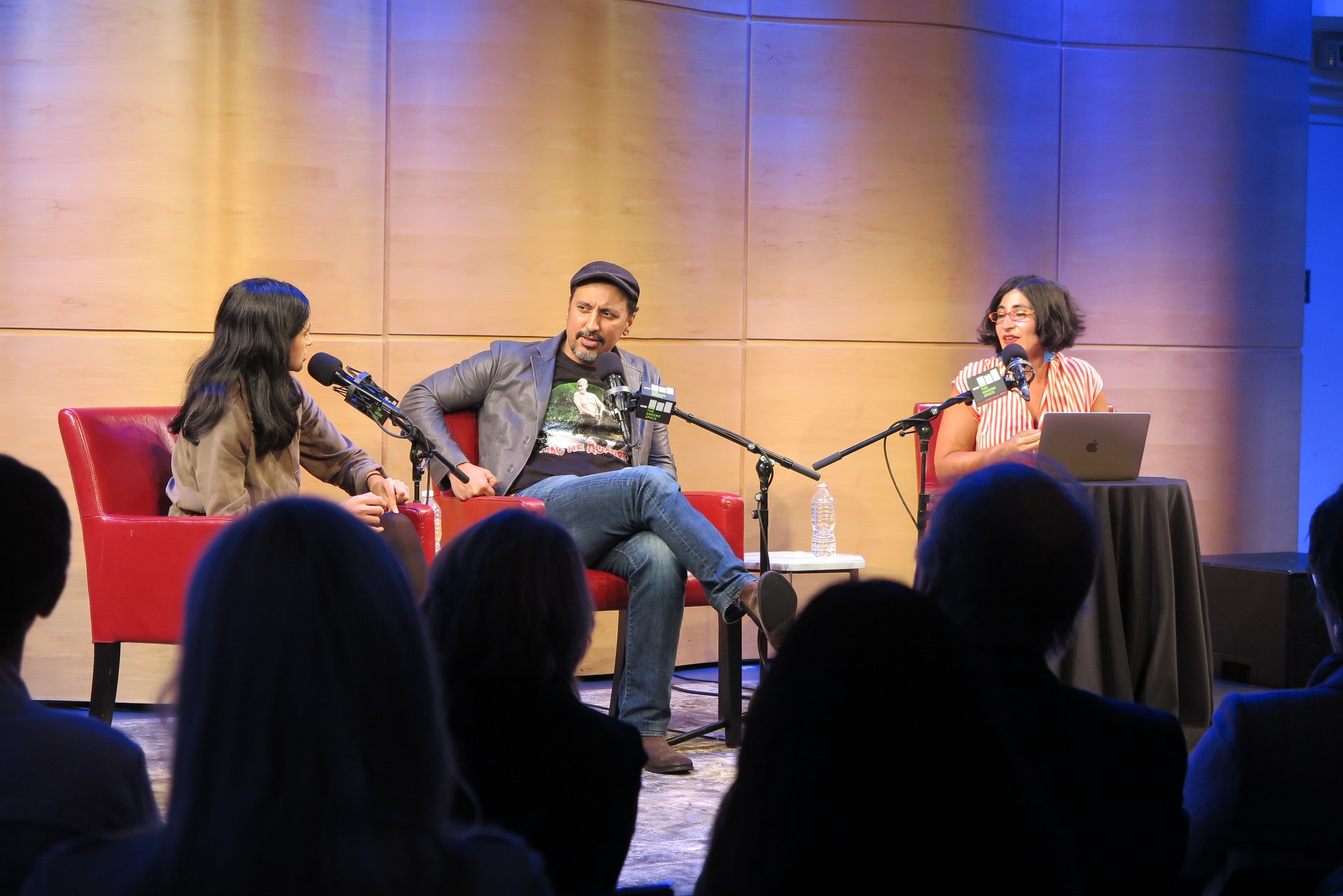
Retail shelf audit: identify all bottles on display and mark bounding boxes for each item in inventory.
[811,482,836,558]
[420,490,441,556]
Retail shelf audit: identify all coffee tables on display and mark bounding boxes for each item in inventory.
[744,552,866,676]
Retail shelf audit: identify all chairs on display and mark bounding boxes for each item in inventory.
[59,407,435,726]
[430,411,744,742]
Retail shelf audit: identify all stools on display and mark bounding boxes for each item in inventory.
[1201,552,1330,688]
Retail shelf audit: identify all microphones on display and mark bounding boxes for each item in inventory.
[1001,343,1031,400]
[595,352,631,446]
[308,352,409,425]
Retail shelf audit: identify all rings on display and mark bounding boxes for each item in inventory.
[1032,450,1036,454]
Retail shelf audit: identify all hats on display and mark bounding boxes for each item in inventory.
[570,261,640,305]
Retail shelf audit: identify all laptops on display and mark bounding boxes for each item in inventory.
[1039,413,1151,480]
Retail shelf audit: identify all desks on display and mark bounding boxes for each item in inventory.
[1044,475,1218,729]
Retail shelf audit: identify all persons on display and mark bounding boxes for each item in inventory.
[397,260,798,774]
[1182,481,1343,896]
[695,462,1187,895]
[934,274,1108,485]
[166,276,429,604]
[0,454,645,896]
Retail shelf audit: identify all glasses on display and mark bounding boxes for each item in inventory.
[988,309,1036,322]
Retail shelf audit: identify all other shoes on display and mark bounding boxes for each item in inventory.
[639,732,695,772]
[738,570,799,651]
